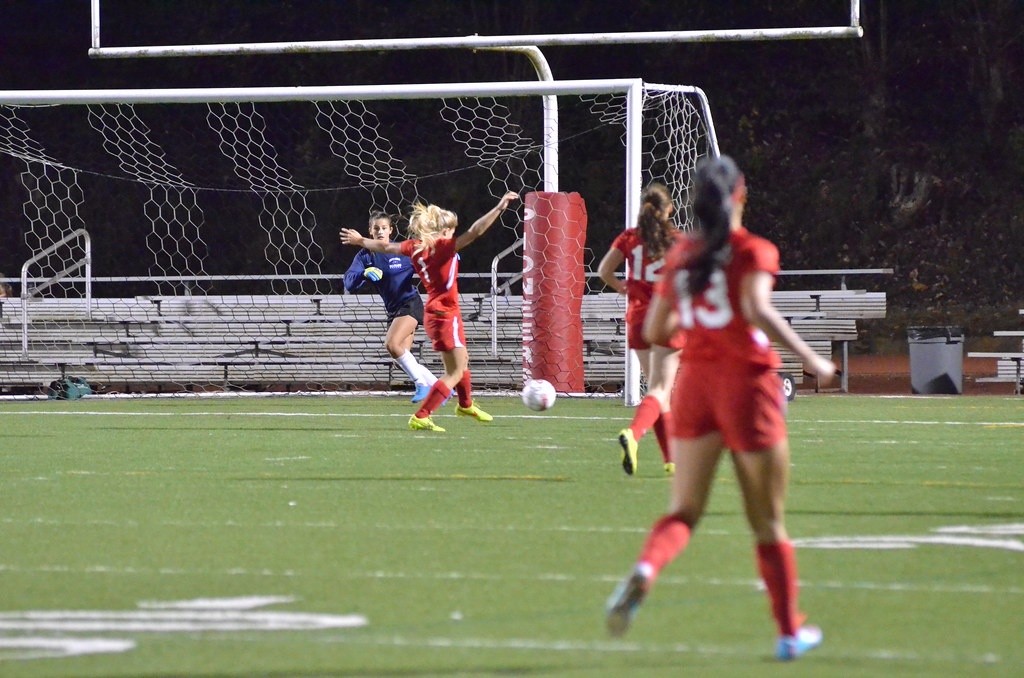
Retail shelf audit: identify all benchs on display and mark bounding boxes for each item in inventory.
[1,289,887,394]
[968,309,1024,395]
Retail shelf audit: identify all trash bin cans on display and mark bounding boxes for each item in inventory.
[906,325,965,394]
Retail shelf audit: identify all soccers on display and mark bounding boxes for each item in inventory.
[521,379,556,411]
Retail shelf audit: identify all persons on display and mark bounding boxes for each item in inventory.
[340,191,521,433]
[597,183,691,477]
[605,155,836,663]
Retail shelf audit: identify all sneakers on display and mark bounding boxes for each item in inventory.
[777,625,824,661]
[408,414,446,433]
[601,564,655,640]
[455,398,493,423]
[441,389,455,407]
[617,428,638,476]
[410,382,431,403]
[663,462,676,476]
